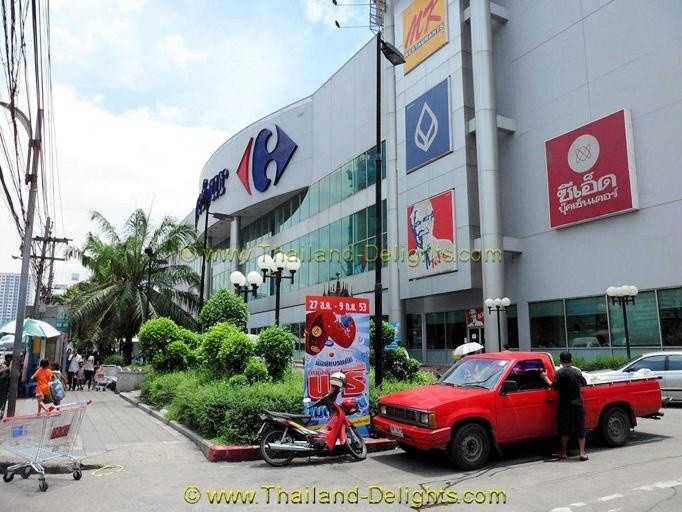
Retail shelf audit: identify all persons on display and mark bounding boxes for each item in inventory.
[468,309,479,324]
[502,344,510,352]
[0,347,101,419]
[541,351,589,460]
[405,200,455,278]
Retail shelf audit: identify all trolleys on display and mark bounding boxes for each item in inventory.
[94,364,143,392]
[1,400,91,492]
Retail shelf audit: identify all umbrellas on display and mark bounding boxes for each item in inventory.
[448,338,482,358]
[1,317,61,352]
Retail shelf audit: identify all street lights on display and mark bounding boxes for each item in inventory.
[199,204,233,307]
[375,32,407,383]
[607,285,638,358]
[259,252,301,327]
[1,102,44,418]
[230,270,263,305]
[12,216,50,317]
[485,297,511,351]
[146,259,168,320]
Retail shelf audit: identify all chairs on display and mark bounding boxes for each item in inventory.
[525,370,542,383]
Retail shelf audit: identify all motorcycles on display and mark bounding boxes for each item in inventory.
[254,371,367,467]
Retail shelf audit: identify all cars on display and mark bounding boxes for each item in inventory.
[589,351,682,406]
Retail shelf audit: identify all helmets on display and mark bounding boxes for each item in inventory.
[330,372,346,388]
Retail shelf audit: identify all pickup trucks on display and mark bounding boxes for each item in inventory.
[371,352,665,469]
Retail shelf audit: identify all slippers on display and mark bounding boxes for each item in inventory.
[579,455,589,461]
